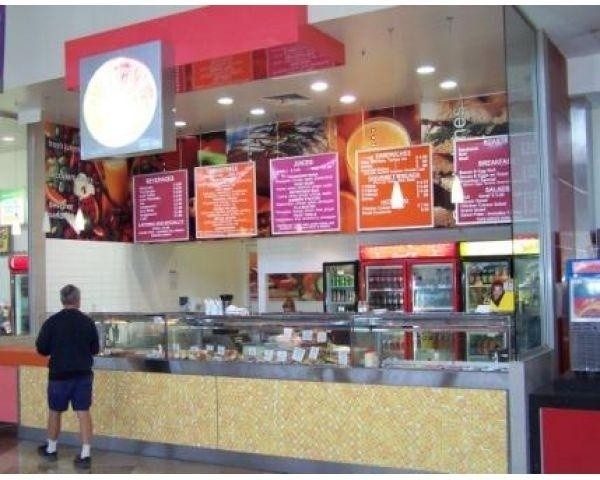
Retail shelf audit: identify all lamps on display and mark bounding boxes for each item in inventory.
[448,99,465,205]
[389,91,406,212]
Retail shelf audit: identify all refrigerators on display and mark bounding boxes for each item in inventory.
[357,241,462,362]
[321,259,359,316]
[460,236,542,362]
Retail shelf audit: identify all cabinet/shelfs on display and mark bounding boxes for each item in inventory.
[17,312,552,474]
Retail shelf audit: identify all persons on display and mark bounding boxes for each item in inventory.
[486,281,514,315]
[280,298,294,314]
[34,283,100,470]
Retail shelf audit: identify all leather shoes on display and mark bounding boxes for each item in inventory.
[38,445,58,462]
[74,455,92,468]
